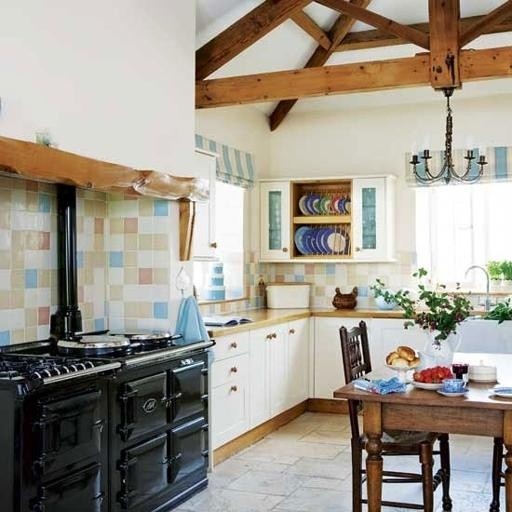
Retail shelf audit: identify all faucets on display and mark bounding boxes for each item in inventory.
[465,265,495,318]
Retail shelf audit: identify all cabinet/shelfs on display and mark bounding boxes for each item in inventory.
[287,318,309,411]
[205,307,249,467]
[258,174,396,262]
[249,323,285,431]
[311,317,416,403]
[1,350,216,512]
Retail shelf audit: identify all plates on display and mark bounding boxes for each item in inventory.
[295,226,350,255]
[299,195,351,215]
[411,381,444,391]
[437,389,469,396]
[487,386,512,398]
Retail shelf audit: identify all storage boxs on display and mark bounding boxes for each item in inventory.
[265,282,311,309]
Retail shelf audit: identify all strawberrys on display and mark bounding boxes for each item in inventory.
[415,364,453,385]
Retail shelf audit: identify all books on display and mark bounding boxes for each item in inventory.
[204,315,253,326]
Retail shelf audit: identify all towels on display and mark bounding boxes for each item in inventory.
[352,376,406,395]
[173,296,215,364]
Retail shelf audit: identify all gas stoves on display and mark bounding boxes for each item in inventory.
[0,327,216,387]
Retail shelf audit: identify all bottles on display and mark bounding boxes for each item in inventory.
[259,275,264,296]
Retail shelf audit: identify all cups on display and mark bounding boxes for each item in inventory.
[442,379,467,392]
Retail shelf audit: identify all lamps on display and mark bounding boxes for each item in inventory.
[410,88,487,185]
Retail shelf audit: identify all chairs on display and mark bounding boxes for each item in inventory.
[339,320,452,512]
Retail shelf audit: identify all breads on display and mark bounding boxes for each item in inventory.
[388,347,420,367]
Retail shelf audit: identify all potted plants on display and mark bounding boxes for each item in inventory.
[502,263,512,292]
[489,262,502,290]
